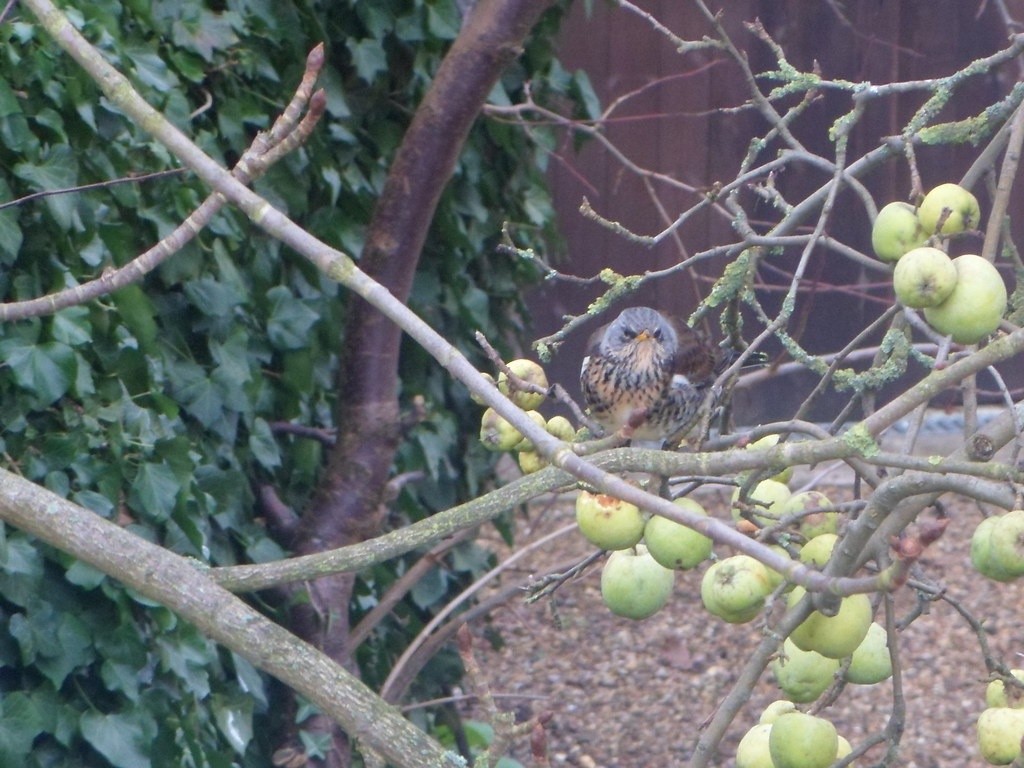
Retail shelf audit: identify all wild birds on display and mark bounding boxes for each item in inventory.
[579,308,729,441]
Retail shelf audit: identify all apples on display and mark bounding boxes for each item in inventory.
[467,184,1023,768]
